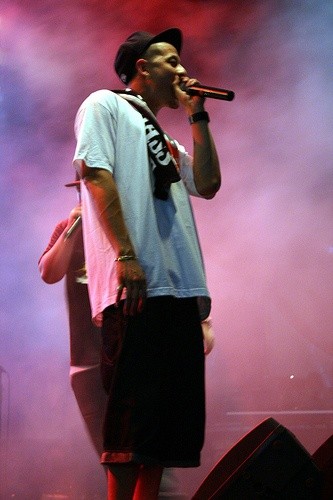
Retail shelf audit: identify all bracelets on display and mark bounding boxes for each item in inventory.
[189,111,210,124]
[113,254,137,263]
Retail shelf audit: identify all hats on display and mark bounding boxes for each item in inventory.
[114,28,183,85]
[64,172,80,187]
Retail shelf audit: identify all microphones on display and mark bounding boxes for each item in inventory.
[65,216,81,238]
[179,82,234,101]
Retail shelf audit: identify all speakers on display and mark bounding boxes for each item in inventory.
[311,434,333,500]
[190,417,322,500]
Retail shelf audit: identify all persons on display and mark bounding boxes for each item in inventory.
[70,26,236,500]
[36,164,126,476]
